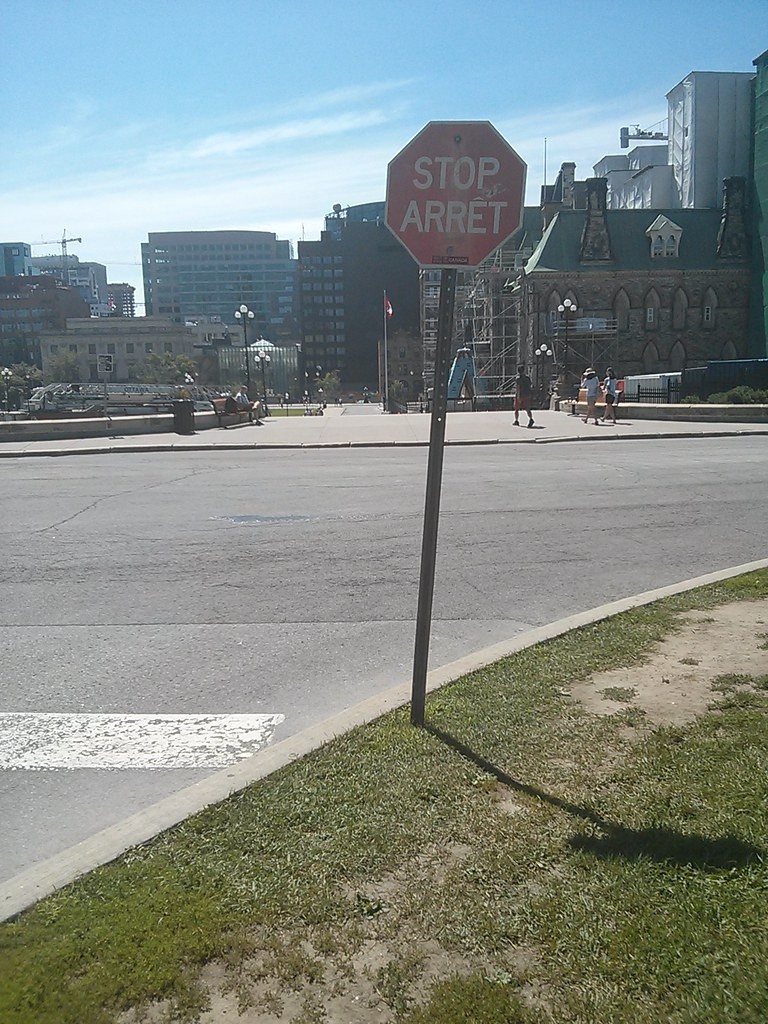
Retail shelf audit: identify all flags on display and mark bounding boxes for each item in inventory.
[386,296,393,317]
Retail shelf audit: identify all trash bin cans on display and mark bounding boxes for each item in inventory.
[172,401,195,435]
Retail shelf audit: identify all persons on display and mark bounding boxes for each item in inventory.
[581,368,621,425]
[512,366,534,427]
[236,386,264,425]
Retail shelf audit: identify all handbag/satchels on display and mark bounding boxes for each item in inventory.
[605,393,615,405]
[596,386,603,400]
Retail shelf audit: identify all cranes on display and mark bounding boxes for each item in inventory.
[619,112,667,148]
[29,228,81,288]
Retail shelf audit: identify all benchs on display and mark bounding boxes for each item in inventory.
[565,388,623,419]
[209,398,254,429]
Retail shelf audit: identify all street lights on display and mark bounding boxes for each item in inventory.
[253,350,272,415]
[305,390,309,413]
[534,344,552,410]
[285,392,289,415]
[318,387,323,409]
[233,304,256,423]
[557,298,577,398]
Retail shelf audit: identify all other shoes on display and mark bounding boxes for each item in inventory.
[592,421,598,425]
[512,421,519,425]
[528,418,534,428]
[581,418,588,424]
[600,417,604,422]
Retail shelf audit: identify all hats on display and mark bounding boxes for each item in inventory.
[583,368,596,375]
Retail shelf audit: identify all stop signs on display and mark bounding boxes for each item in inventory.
[382,120,528,269]
